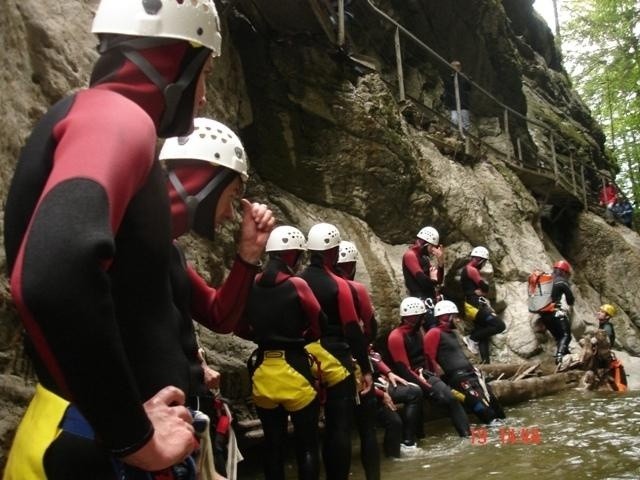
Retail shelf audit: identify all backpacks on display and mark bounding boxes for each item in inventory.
[525,270,560,314]
[601,361,629,393]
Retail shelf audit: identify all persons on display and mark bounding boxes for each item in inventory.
[154,114,276,480]
[0,0,226,478]
[594,301,628,392]
[620,197,632,229]
[444,57,473,132]
[597,171,620,210]
[528,259,577,365]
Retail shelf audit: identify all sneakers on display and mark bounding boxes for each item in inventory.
[400,442,425,455]
[462,334,481,356]
[554,352,564,365]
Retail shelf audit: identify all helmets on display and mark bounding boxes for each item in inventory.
[599,304,619,318]
[336,239,359,264]
[416,226,441,248]
[89,0,223,59]
[398,296,427,316]
[551,260,571,274]
[432,298,460,318]
[157,116,250,199]
[470,245,491,261]
[264,225,308,253]
[479,261,495,274]
[307,223,342,251]
